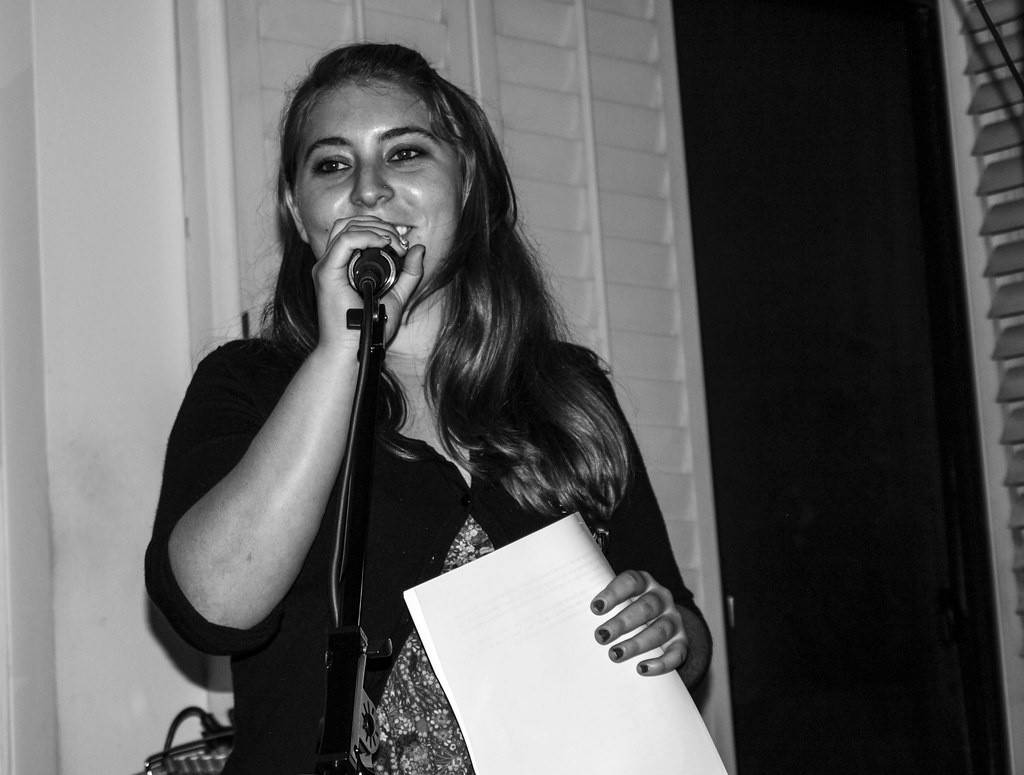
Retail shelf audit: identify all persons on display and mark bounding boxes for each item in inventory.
[143,44,713,775]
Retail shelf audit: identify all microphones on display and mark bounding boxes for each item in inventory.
[347,244,402,298]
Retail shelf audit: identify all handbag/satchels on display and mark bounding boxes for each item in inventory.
[144,707,236,775]
